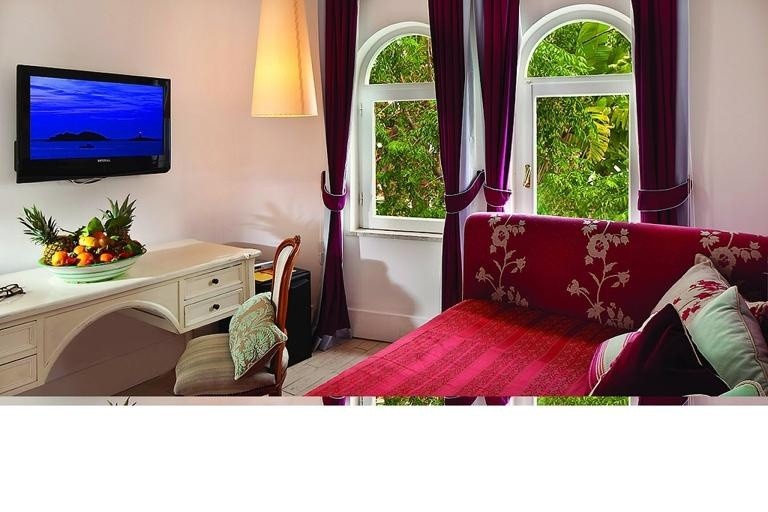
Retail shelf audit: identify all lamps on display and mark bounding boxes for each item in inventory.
[250,0,323,122]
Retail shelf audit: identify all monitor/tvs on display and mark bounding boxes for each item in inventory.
[13,62,172,185]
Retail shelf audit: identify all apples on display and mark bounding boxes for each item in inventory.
[52,241,142,266]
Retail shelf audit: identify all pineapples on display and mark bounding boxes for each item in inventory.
[97,193,137,244]
[17,204,74,266]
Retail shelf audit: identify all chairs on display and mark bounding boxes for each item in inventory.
[172,233,303,399]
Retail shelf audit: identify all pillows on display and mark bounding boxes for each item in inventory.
[224,285,289,382]
[574,254,768,406]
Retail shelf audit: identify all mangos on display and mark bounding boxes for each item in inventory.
[79,232,110,248]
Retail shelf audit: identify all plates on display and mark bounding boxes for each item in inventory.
[39,247,147,282]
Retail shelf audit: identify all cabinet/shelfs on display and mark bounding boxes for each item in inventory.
[0,238,262,397]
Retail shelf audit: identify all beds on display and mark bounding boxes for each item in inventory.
[310,205,766,407]
